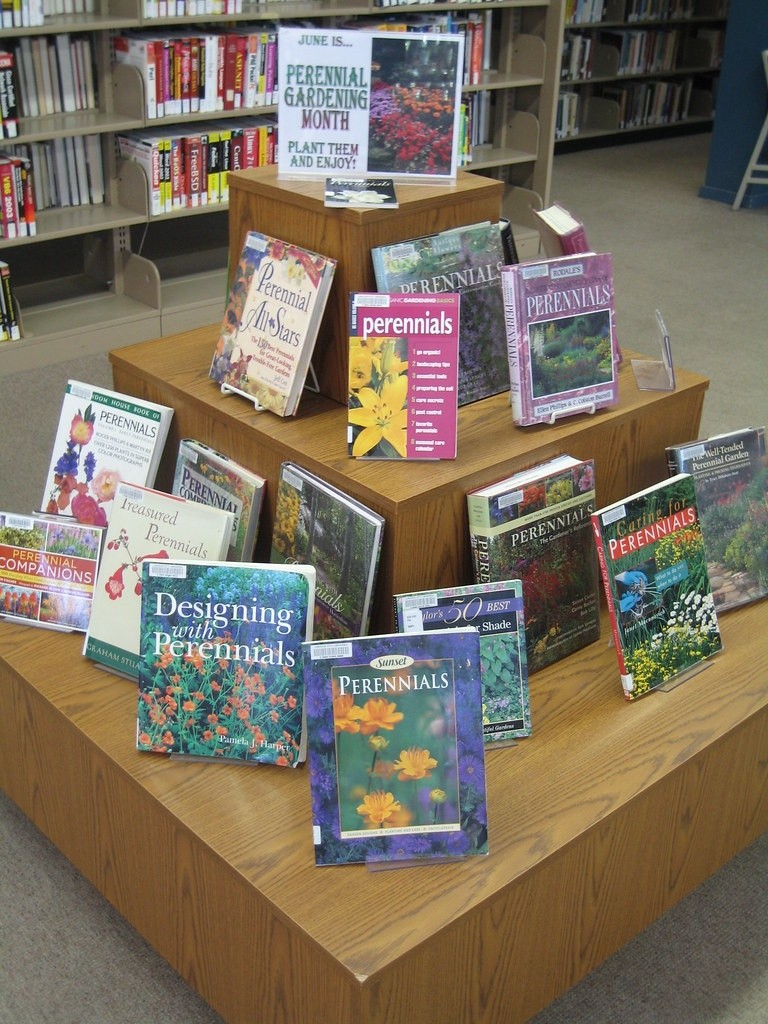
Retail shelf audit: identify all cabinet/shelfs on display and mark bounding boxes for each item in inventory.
[0,0,568,375]
[553,0,729,148]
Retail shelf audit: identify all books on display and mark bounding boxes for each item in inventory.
[303,626,488,869]
[554,0,723,140]
[394,581,532,747]
[530,201,591,260]
[41,375,173,533]
[208,228,335,419]
[1,2,498,339]
[173,436,267,559]
[132,556,313,771]
[662,430,767,613]
[324,177,398,210]
[497,217,520,264]
[346,291,458,461]
[273,459,384,639]
[500,251,620,430]
[577,474,726,702]
[83,481,234,692]
[371,222,513,406]
[473,453,601,678]
